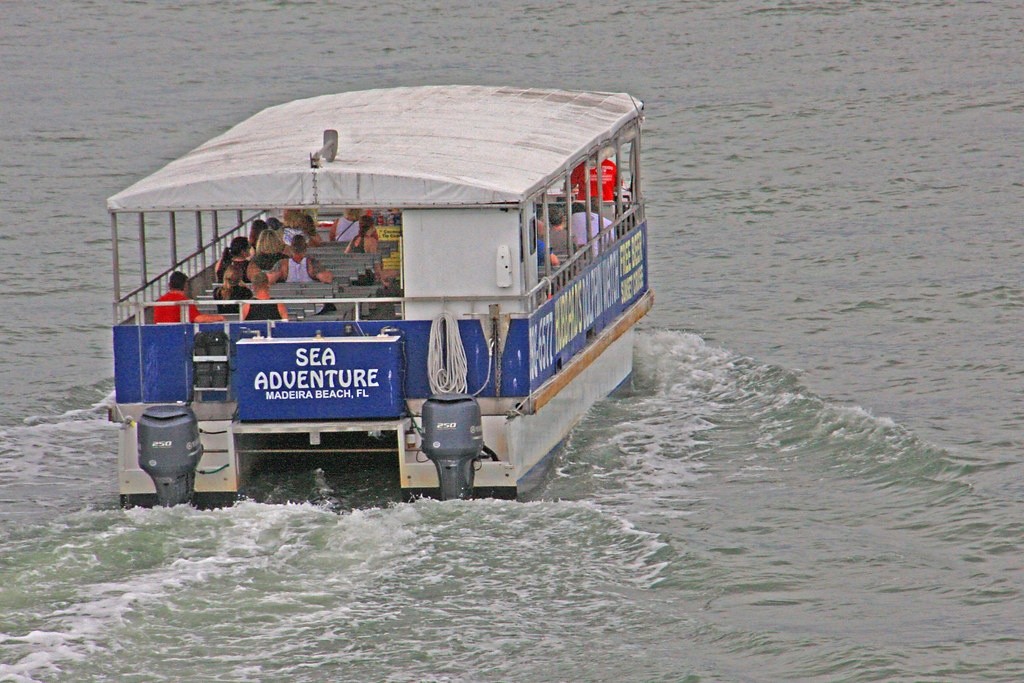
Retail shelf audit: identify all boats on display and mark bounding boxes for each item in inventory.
[104,83,656,501]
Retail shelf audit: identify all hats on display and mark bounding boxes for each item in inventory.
[548,206,564,219]
[170,271,188,284]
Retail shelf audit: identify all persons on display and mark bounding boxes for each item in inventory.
[526,150,623,268]
[154,272,224,322]
[345,215,377,254]
[154,208,378,323]
[266,235,333,283]
[240,272,287,320]
